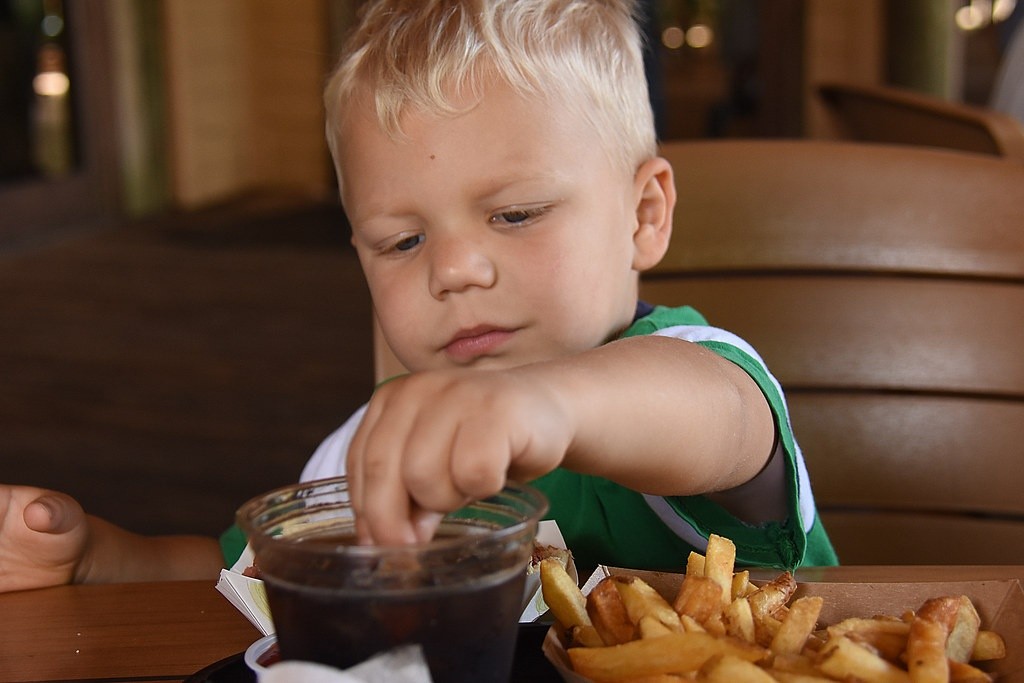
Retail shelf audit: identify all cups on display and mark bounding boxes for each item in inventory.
[234,477,550,682]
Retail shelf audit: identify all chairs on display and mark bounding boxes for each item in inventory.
[374,80,1024,566]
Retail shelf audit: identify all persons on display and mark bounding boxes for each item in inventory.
[0,0,839,593]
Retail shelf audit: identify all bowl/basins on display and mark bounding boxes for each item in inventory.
[245,631,284,679]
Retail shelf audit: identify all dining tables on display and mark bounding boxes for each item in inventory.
[0,566,1023,683]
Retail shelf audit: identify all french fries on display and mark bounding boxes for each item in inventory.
[540,533,1004,683]
